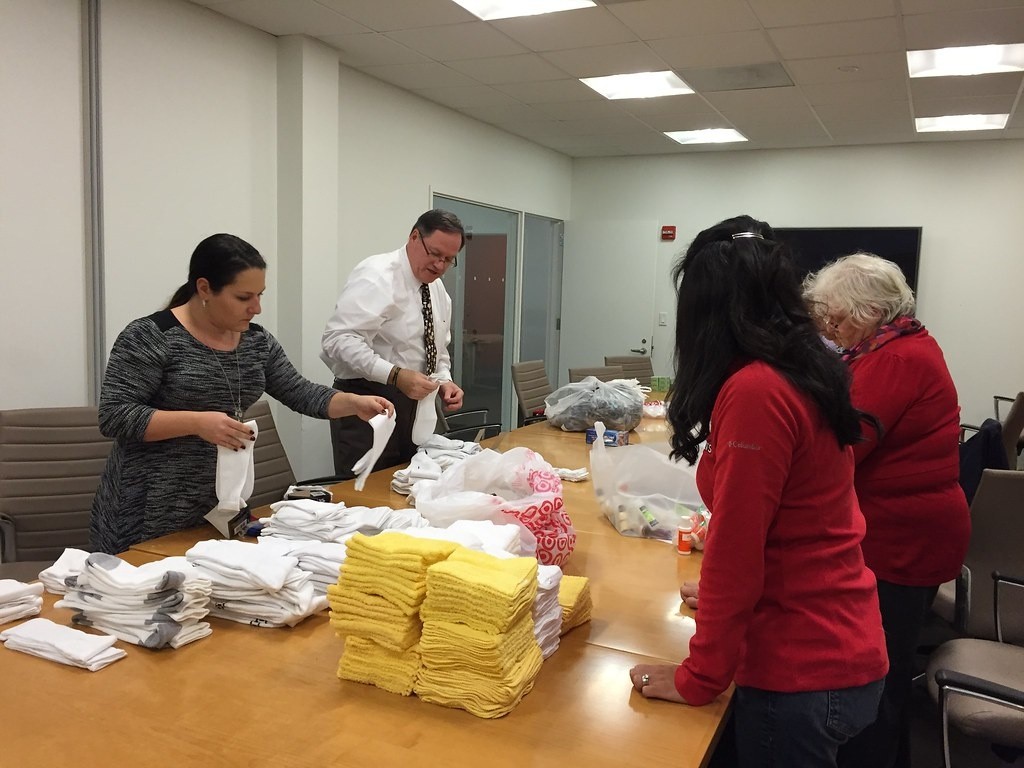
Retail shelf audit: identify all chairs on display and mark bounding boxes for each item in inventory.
[510,359,554,426]
[230,401,356,515]
[951,419,1009,507]
[604,356,655,389]
[1,407,123,562]
[928,469,1024,649]
[435,394,502,443]
[568,365,625,385]
[923,572,1024,768]
[960,392,1024,479]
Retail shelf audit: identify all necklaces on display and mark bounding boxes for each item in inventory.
[189,300,243,425]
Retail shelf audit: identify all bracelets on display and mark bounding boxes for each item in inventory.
[392,367,401,386]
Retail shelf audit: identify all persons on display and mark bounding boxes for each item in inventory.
[1001,392,1024,473]
[319,209,465,481]
[627,214,889,768]
[88,232,395,556]
[804,251,974,768]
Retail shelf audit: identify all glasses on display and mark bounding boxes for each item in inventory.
[824,310,852,332]
[418,229,457,267]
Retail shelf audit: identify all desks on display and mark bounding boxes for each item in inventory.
[0,390,759,768]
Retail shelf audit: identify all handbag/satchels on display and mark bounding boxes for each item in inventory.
[588,419,714,539]
[410,447,576,577]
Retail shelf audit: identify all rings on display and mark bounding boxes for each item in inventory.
[642,673,649,686]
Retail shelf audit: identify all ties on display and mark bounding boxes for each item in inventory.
[420,283,437,376]
